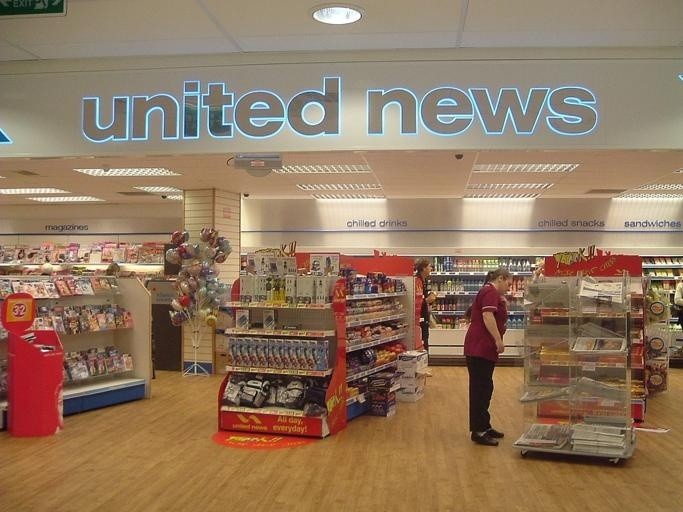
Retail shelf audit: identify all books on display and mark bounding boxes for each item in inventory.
[512,272,636,462]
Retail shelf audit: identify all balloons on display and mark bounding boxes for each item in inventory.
[164,225,233,332]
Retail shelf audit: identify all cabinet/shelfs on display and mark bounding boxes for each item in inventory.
[1,246,179,284]
[641,257,683,362]
[1,273,152,423]
[416,256,538,363]
[513,278,630,458]
[339,272,414,411]
[524,277,646,422]
[217,274,345,430]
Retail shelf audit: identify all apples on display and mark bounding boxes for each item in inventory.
[374,342,405,365]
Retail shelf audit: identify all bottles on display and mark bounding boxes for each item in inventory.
[427,256,530,329]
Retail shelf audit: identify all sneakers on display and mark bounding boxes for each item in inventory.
[470,428,505,447]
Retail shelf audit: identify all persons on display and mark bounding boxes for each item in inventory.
[463,267,515,446]
[673,281,682,333]
[68,319,80,336]
[412,258,437,377]
[54,318,64,333]
